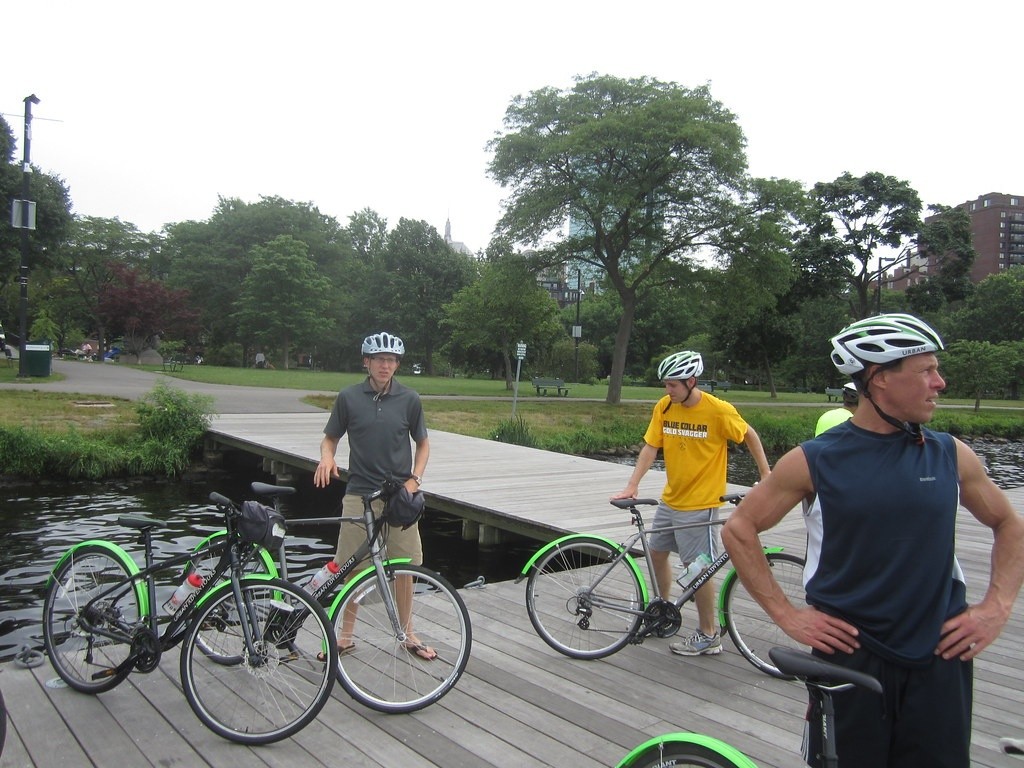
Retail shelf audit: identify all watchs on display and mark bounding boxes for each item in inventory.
[410,475,422,486]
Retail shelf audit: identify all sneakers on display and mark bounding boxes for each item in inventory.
[626,622,651,636]
[669,628,723,655]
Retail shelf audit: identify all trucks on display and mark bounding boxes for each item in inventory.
[0,323,5,351]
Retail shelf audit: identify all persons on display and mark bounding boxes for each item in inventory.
[721,314,1024,768]
[609,350,772,656]
[815,382,859,437]
[83,342,92,358]
[313,332,439,661]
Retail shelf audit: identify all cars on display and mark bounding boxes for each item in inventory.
[56,347,74,358]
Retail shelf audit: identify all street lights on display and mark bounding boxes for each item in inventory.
[574,266,581,385]
[17,94,43,377]
[876,256,897,316]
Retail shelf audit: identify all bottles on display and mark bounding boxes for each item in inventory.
[164,574,204,614]
[676,553,711,587]
[302,561,340,595]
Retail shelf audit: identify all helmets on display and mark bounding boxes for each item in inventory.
[842,381,858,393]
[828,313,944,375]
[360,332,406,356]
[657,350,704,380]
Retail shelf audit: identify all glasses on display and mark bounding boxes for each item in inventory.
[367,357,400,364]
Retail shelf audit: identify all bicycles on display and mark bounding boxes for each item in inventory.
[43,476,472,746]
[616,646,1024,768]
[513,492,821,681]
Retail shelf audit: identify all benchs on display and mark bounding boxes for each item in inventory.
[697,379,717,396]
[825,389,845,402]
[531,378,571,397]
[163,358,185,371]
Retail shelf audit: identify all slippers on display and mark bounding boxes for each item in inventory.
[400,641,438,660]
[316,643,356,661]
